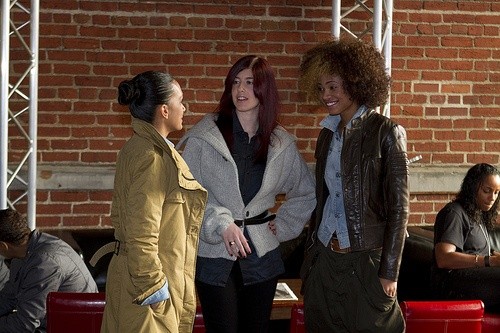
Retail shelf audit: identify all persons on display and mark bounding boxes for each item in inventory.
[174,53,318,333]
[432,162,500,275]
[0,206,102,333]
[103,68,209,333]
[298,40,410,333]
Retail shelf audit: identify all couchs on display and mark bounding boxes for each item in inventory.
[82,226,446,298]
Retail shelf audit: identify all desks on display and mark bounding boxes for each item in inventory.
[196,279,303,320]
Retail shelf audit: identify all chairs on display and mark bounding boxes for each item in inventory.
[46,292,203,333]
[289,299,500,333]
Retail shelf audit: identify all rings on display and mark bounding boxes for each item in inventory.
[228,241,236,247]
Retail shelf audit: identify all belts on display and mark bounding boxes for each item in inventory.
[234,210,276,228]
[329,237,351,254]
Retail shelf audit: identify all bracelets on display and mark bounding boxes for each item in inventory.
[483,255,490,268]
[474,254,479,268]
